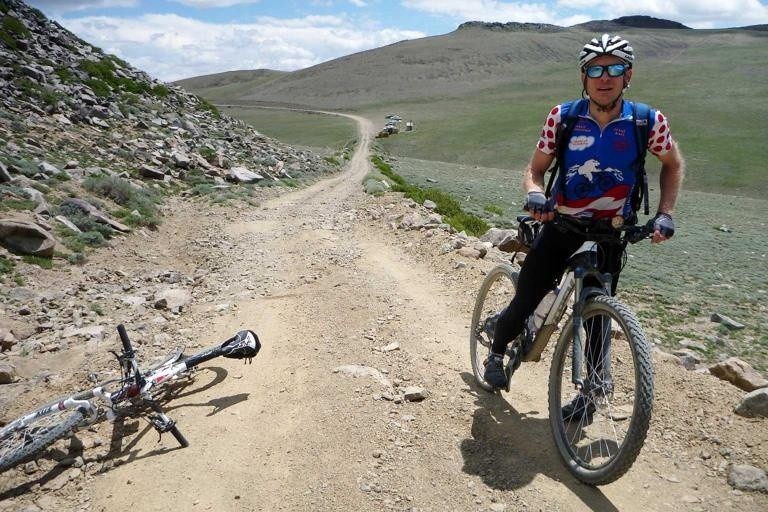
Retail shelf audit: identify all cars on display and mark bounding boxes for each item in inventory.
[379,114,403,136]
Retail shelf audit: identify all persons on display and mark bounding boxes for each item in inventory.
[482,34,685,424]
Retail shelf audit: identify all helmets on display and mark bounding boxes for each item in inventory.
[576,33,635,69]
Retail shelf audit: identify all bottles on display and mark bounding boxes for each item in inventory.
[533,285,561,330]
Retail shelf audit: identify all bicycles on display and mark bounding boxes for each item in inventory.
[468,200,657,486]
[0,320,264,475]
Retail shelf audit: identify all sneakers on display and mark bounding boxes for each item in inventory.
[483,345,508,388]
[561,394,596,421]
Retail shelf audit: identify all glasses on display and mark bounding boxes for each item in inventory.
[584,63,630,78]
